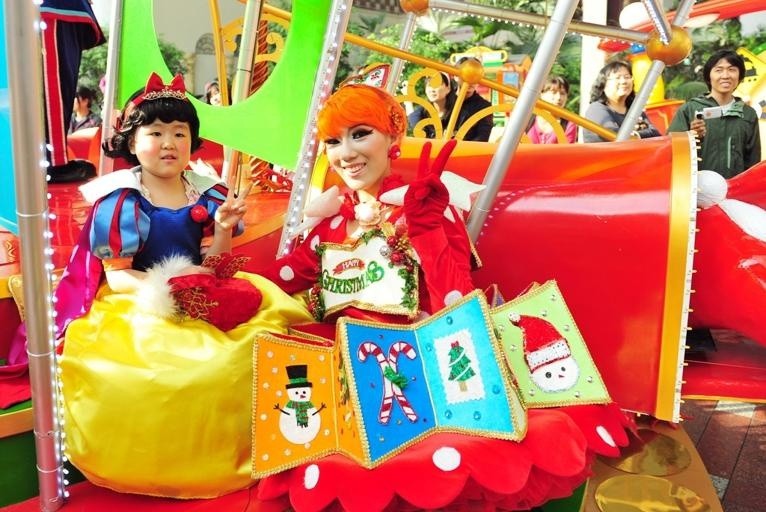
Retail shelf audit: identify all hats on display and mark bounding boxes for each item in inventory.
[285,364,313,390]
[508,312,572,374]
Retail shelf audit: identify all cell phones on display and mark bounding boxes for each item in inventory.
[696,112,704,119]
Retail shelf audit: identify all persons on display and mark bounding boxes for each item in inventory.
[203,78,231,107]
[67,85,103,135]
[9,72,316,500]
[256,61,647,512]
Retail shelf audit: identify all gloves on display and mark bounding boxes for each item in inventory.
[403,138,458,241]
